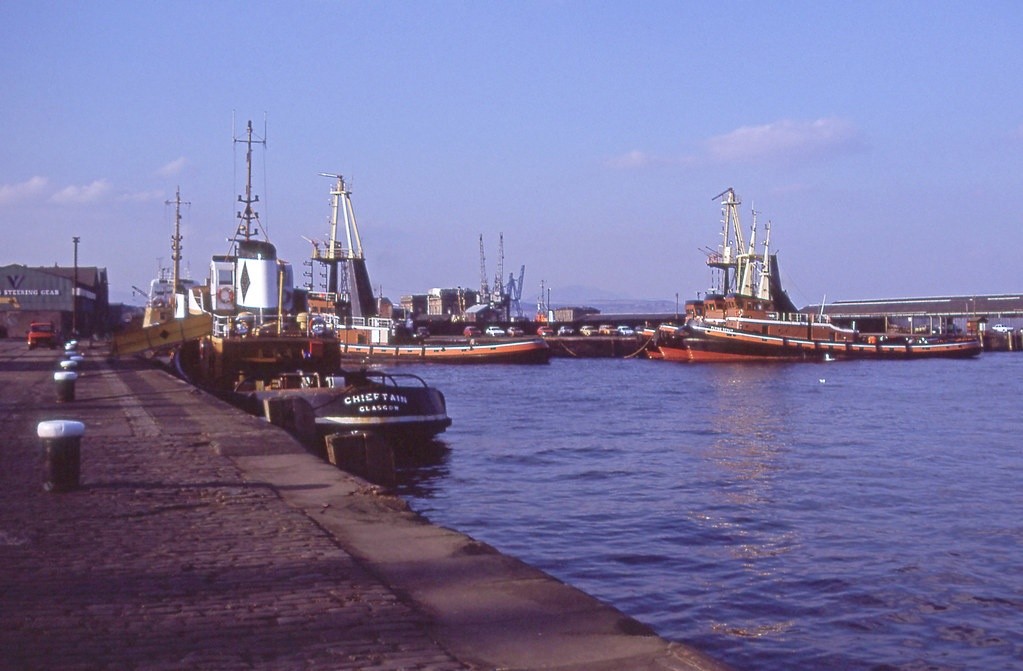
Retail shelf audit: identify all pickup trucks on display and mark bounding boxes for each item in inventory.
[915,324,930,333]
[992,324,1014,333]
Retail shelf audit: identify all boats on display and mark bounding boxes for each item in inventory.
[645,188,981,362]
[132,109,551,454]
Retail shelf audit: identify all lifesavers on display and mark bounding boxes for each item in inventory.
[309,317,326,335]
[218,287,234,303]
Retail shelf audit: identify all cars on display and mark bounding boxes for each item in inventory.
[538,325,651,337]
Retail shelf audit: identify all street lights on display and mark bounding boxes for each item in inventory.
[72,237,80,338]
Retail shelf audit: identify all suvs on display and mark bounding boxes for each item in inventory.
[463,326,481,337]
[486,327,505,337]
[507,327,524,337]
[416,327,430,339]
[26,320,59,350]
